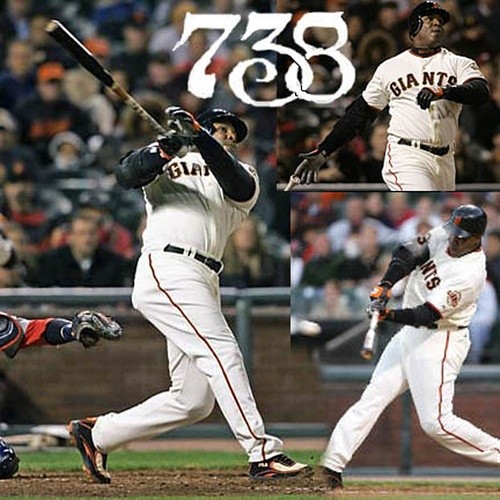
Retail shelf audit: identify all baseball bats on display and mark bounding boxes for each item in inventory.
[45,19,188,158]
[360,308,380,361]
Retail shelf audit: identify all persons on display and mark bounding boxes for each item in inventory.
[38,200,133,257]
[398,196,443,244]
[0,310,122,480]
[317,189,500,203]
[291,191,398,283]
[293,1,500,191]
[67,107,316,484]
[483,227,500,307]
[317,204,500,489]
[308,276,355,323]
[290,190,316,354]
[218,212,288,287]
[465,278,500,364]
[444,191,499,226]
[67,0,293,107]
[36,205,128,287]
[0,0,67,288]
[67,109,133,204]
[415,220,435,235]
[234,107,292,210]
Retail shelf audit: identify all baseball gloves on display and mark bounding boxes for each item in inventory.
[71,310,123,348]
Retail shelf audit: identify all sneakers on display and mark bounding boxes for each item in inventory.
[308,465,341,491]
[67,415,112,483]
[248,452,315,477]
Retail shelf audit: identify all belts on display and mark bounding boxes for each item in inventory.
[421,322,471,330]
[164,244,222,274]
[397,137,450,157]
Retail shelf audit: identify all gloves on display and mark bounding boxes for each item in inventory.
[367,301,390,324]
[157,119,197,158]
[368,285,393,303]
[416,85,445,110]
[292,150,328,185]
[166,104,205,146]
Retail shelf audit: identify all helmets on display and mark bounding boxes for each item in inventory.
[194,108,248,145]
[441,204,487,240]
[408,0,452,37]
